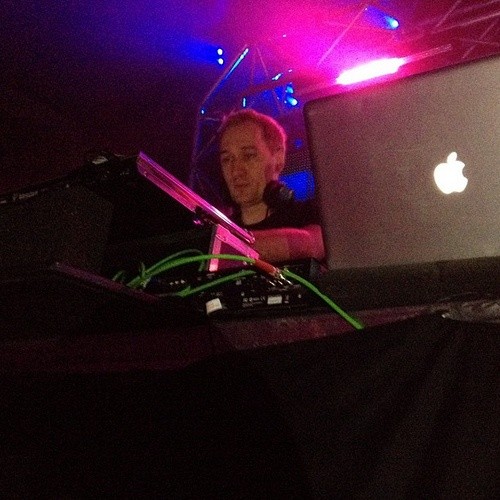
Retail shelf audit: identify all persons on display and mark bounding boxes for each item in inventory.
[185,110,326,272]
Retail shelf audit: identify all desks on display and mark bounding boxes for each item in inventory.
[0,297,500,500]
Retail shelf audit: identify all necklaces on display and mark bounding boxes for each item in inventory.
[263,206,271,229]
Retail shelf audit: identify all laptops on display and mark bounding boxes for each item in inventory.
[303,52,500,273]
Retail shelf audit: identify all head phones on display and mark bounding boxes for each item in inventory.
[262,180,296,209]
